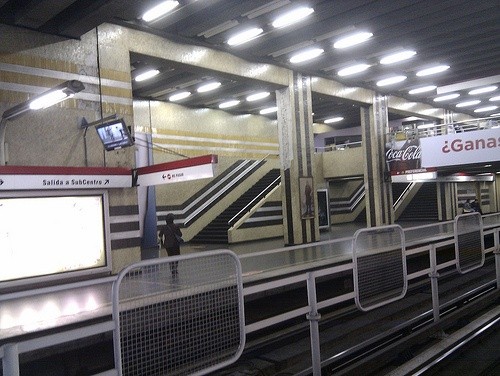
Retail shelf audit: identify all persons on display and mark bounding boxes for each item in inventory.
[159,213,183,273]
[465,199,485,218]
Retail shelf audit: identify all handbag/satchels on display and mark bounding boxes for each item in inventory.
[166,225,184,244]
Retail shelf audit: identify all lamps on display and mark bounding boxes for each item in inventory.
[0,80,86,165]
